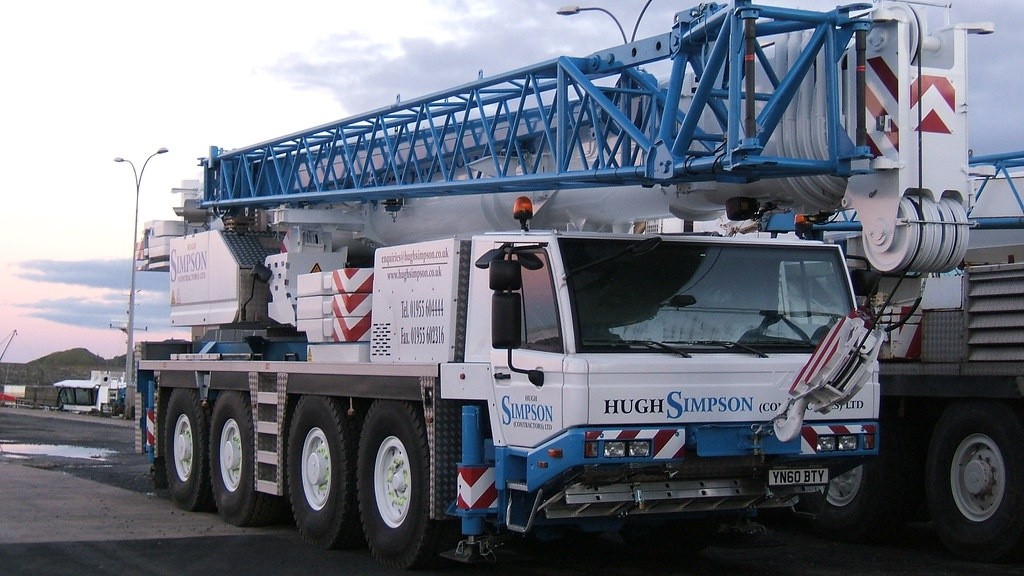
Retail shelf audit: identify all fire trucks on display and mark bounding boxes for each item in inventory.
[609,149,1024,560]
[139,0,979,569]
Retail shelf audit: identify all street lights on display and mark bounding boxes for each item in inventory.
[114,147,169,418]
[556,0,653,72]
